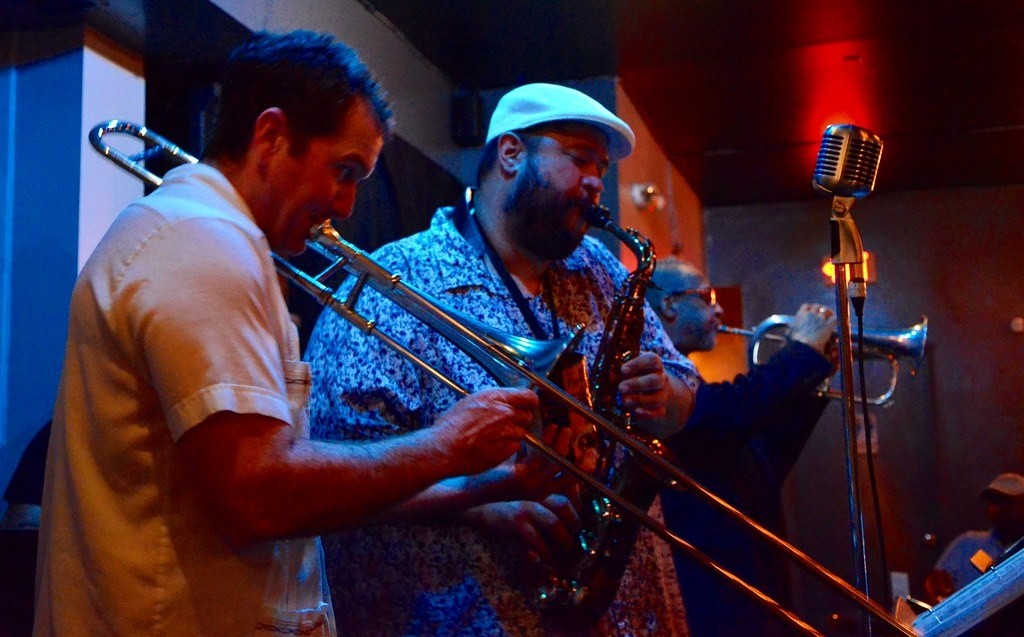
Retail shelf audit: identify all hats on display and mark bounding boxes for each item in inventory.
[988,472,1024,496]
[484,81,638,163]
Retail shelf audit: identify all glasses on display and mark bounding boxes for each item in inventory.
[981,489,1017,507]
[664,285,721,308]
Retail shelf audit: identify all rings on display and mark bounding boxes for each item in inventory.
[819,308,827,313]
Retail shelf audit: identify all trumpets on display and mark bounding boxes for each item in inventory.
[713,305,933,413]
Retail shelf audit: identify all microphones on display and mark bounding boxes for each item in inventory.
[811,124,885,315]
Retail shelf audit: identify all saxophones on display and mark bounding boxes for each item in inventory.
[505,197,688,630]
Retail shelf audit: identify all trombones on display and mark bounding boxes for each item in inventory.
[83,111,928,637]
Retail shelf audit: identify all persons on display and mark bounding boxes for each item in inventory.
[645,261,840,637]
[302,83,699,637]
[34,27,596,637]
[925,473,1024,637]
